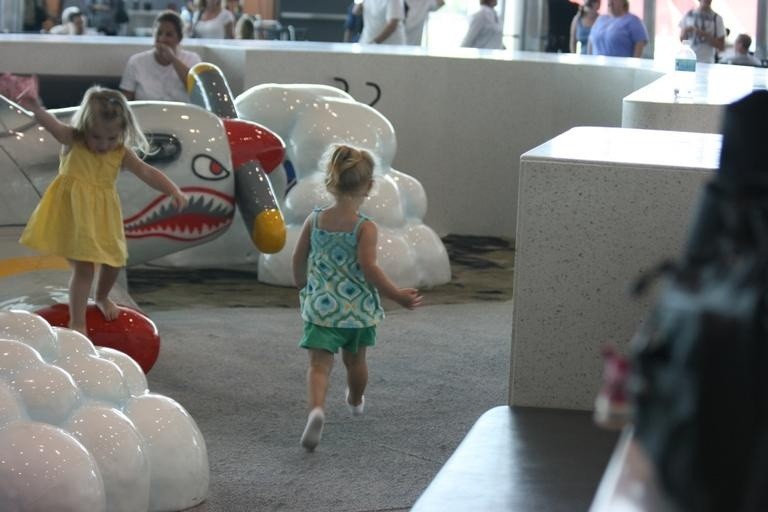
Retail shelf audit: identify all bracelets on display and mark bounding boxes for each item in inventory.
[370,39,379,45]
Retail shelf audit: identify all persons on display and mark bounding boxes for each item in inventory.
[403,1,445,45]
[544,0,581,53]
[290,143,421,449]
[570,1,601,54]
[120,11,204,105]
[678,0,725,63]
[352,1,407,44]
[39,1,257,42]
[460,1,505,50]
[342,3,363,45]
[720,33,762,65]
[586,1,648,57]
[18,88,186,340]
